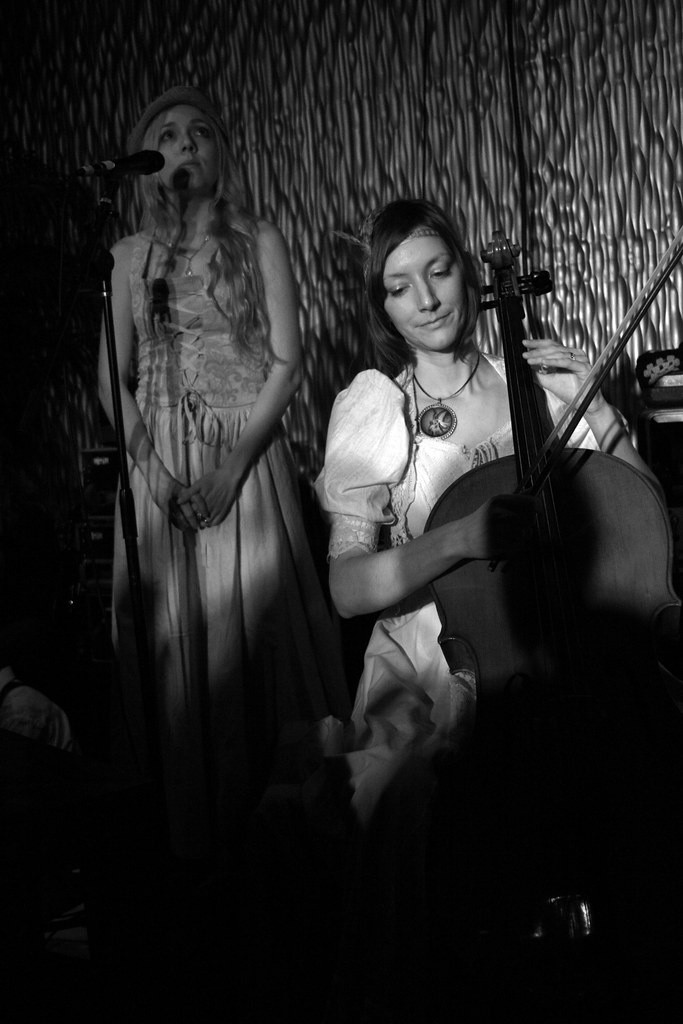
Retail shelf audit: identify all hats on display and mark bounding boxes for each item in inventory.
[127,87,231,157]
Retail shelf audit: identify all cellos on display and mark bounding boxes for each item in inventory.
[424,227,683,1000]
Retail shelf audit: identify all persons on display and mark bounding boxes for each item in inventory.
[312,199,667,832]
[97,87,351,790]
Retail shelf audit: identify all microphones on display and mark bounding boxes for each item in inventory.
[78,149,165,176]
